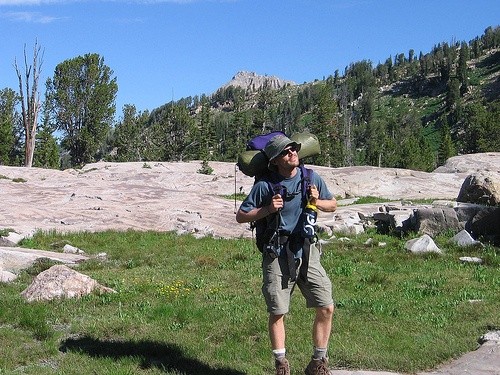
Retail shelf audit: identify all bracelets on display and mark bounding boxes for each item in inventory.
[265,206,271,215]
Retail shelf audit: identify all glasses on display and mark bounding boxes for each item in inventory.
[274,147,297,159]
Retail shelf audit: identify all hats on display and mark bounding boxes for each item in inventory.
[264,135,301,171]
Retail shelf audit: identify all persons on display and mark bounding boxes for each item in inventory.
[236,134,337,375]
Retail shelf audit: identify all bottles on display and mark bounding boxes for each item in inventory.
[300,205,317,237]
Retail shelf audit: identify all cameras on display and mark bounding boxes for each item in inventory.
[267,246,279,258]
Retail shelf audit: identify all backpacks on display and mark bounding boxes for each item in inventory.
[249,131,313,253]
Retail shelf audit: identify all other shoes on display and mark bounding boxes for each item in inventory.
[274,359,291,375]
[304,358,332,375]
